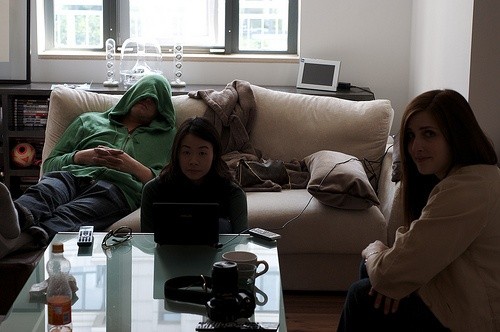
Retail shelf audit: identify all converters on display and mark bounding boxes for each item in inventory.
[338,82,350,91]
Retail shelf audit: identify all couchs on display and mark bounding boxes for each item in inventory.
[39,86,408,294]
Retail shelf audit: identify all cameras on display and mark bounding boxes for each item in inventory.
[206,260,255,322]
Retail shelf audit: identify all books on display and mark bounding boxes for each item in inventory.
[9,98,49,128]
[10,128,44,160]
[16,177,39,193]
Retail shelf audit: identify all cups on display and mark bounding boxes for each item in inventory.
[221,251,269,280]
[235,262,256,292]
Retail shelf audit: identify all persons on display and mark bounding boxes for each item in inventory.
[142,117,248,246]
[336,90,500,332]
[0,74,179,252]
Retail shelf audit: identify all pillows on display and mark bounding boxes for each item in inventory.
[302,149,381,212]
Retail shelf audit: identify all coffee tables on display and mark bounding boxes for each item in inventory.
[0,231,287,332]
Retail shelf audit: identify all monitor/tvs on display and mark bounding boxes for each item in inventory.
[296,58,341,91]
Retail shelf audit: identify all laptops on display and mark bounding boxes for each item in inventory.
[152,202,220,246]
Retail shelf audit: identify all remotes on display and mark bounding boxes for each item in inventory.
[249,227,281,241]
[196,321,280,332]
[77,225,94,248]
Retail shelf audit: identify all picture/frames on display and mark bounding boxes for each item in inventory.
[296,58,341,92]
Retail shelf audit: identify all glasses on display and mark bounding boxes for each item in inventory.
[102,226,133,246]
[105,242,134,260]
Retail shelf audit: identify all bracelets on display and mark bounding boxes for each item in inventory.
[363,251,378,266]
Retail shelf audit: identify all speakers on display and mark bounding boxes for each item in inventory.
[104,39,118,86]
[170,44,186,87]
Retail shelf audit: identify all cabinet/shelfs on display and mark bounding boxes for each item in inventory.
[0,82,375,201]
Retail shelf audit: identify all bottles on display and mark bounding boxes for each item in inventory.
[46,243,73,332]
